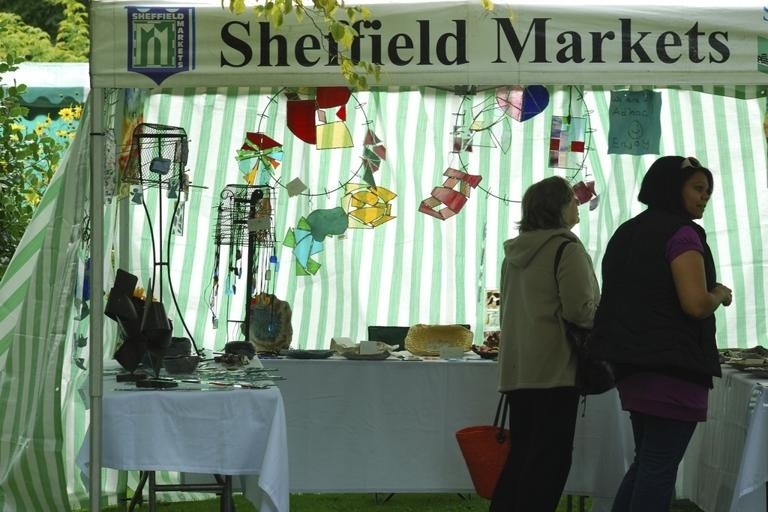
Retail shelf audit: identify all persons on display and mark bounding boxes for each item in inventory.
[489,177,600,512]
[594,156,732,512]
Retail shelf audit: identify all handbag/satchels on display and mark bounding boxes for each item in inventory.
[456,425,509,500]
[565,325,615,396]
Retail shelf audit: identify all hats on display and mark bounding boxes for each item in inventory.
[404,324,474,356]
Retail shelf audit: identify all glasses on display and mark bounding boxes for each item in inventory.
[681,157,700,169]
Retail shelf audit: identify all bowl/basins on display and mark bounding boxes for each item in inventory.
[162,354,199,375]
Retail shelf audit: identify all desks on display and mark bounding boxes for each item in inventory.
[88,352,768,512]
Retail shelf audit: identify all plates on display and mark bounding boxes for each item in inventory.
[290,349,336,359]
[725,361,768,378]
